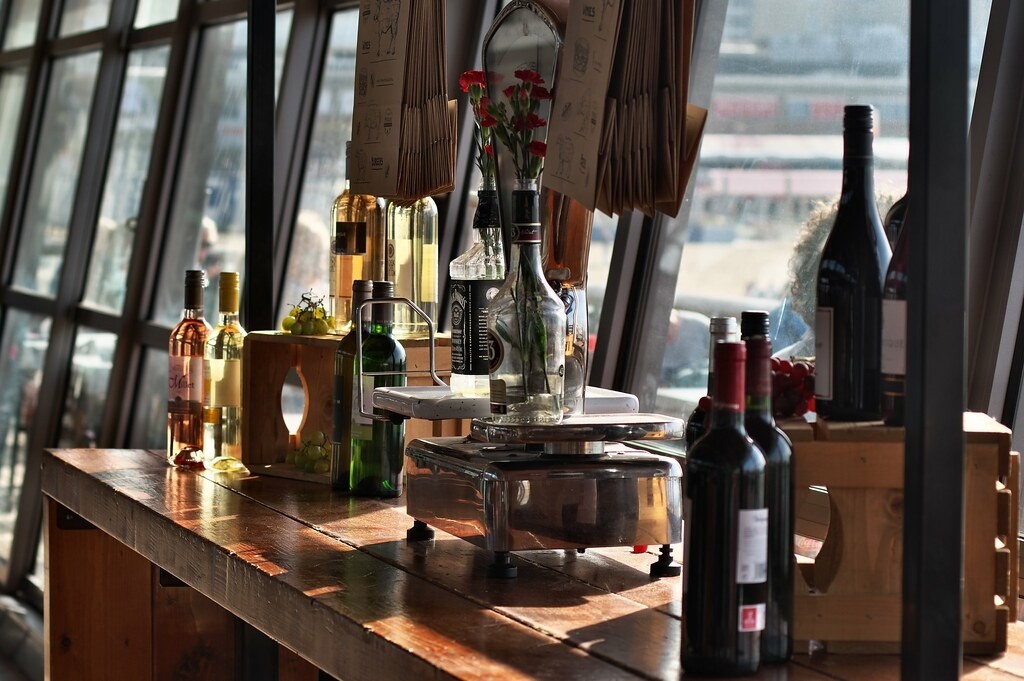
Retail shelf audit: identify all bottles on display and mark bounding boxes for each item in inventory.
[813,103,893,421]
[736,310,797,666]
[388,199,437,338]
[879,178,907,426]
[206,270,252,472]
[167,270,213,469]
[327,279,374,494]
[449,190,506,396]
[684,318,739,464]
[680,337,769,681]
[347,280,407,498]
[486,189,565,423]
[330,140,384,335]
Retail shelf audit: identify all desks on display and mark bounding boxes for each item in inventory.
[38,445,1024,681]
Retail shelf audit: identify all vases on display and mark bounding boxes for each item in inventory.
[487,179,566,425]
[449,179,506,396]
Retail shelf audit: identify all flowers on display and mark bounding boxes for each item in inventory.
[478,67,547,182]
[459,70,496,179]
